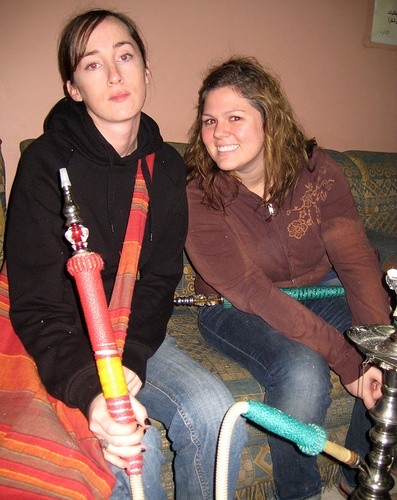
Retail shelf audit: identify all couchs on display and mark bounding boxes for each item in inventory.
[19,139,397,500]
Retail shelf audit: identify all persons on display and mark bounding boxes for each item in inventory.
[3,11,245,499]
[185,60,392,500]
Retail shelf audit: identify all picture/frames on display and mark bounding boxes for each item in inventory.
[363,0,397,51]
